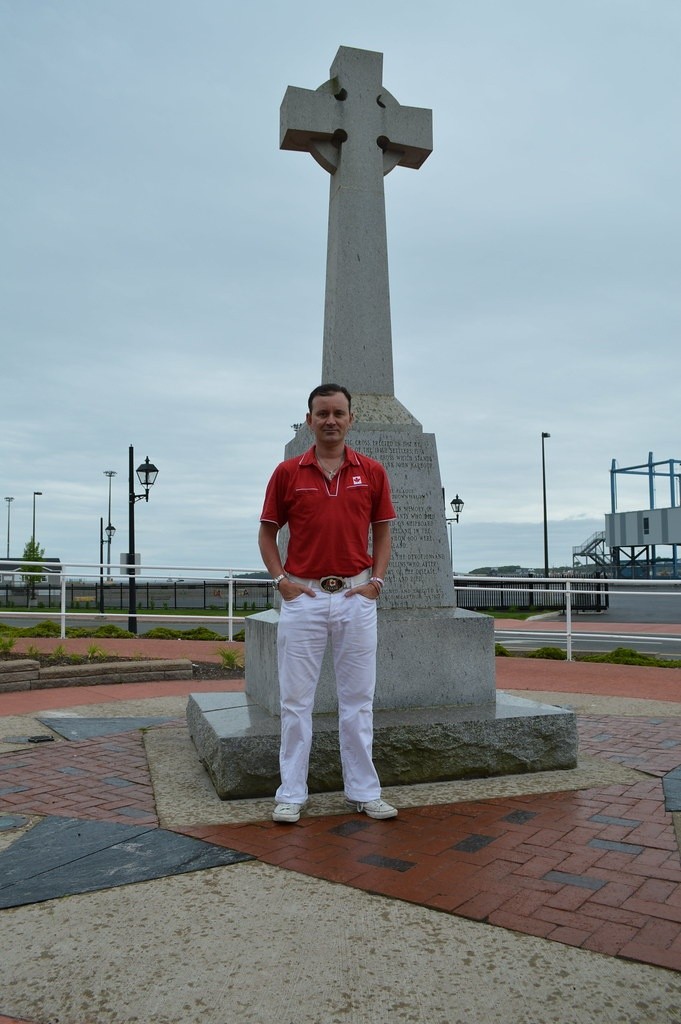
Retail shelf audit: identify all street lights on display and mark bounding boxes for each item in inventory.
[540,432,551,589]
[128,456,159,640]
[98,523,116,614]
[447,522,453,569]
[5,496,14,558]
[104,470,116,582]
[31,491,42,600]
[444,495,463,530]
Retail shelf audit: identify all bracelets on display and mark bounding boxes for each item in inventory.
[369,577,384,599]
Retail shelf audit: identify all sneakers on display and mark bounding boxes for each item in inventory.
[345,798,398,820]
[272,800,307,823]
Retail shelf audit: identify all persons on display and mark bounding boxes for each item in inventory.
[258,385,397,823]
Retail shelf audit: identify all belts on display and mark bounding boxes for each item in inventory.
[289,569,371,593]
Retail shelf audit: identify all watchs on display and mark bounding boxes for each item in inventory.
[272,574,286,590]
